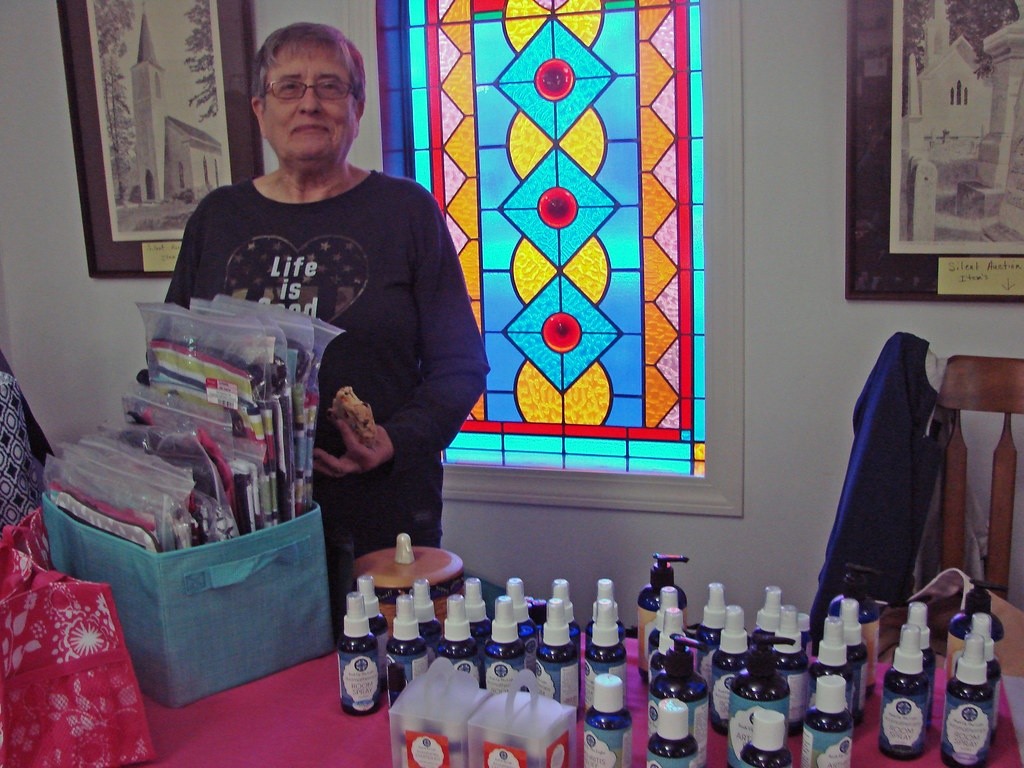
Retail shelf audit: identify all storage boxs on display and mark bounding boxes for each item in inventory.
[39,490,327,706]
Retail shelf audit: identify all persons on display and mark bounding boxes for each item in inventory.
[149,22,491,585]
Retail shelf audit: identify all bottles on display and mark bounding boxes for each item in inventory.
[336,591,383,717]
[356,574,390,691]
[386,553,1006,768]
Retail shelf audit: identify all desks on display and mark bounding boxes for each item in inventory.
[132,662,1022,768]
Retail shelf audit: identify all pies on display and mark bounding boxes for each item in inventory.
[326,387,377,447]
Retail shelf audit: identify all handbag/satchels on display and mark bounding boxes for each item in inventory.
[0,505,157,768]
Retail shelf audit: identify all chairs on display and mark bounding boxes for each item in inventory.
[890,332,1024,622]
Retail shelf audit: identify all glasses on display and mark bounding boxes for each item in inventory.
[264,78,356,100]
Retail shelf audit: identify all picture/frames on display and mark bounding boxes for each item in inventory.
[54,1,265,280]
[842,0,1024,303]
